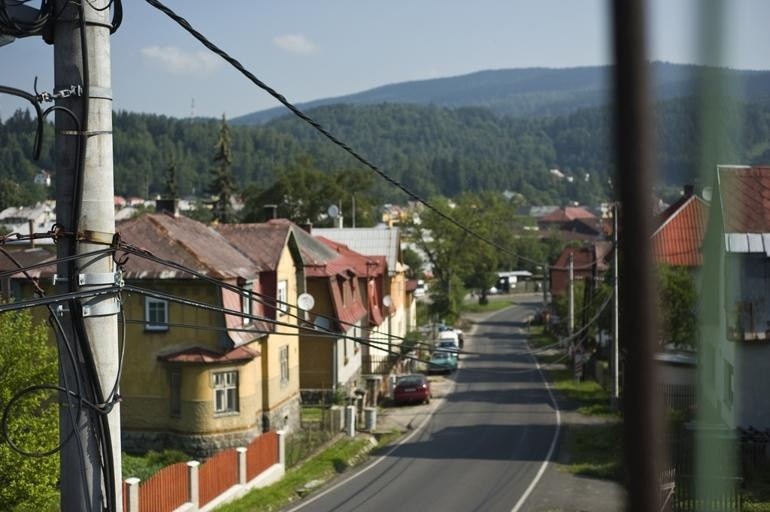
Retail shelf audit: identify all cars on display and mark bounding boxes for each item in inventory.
[394,374,431,406]
[427,348,460,372]
[418,322,465,350]
